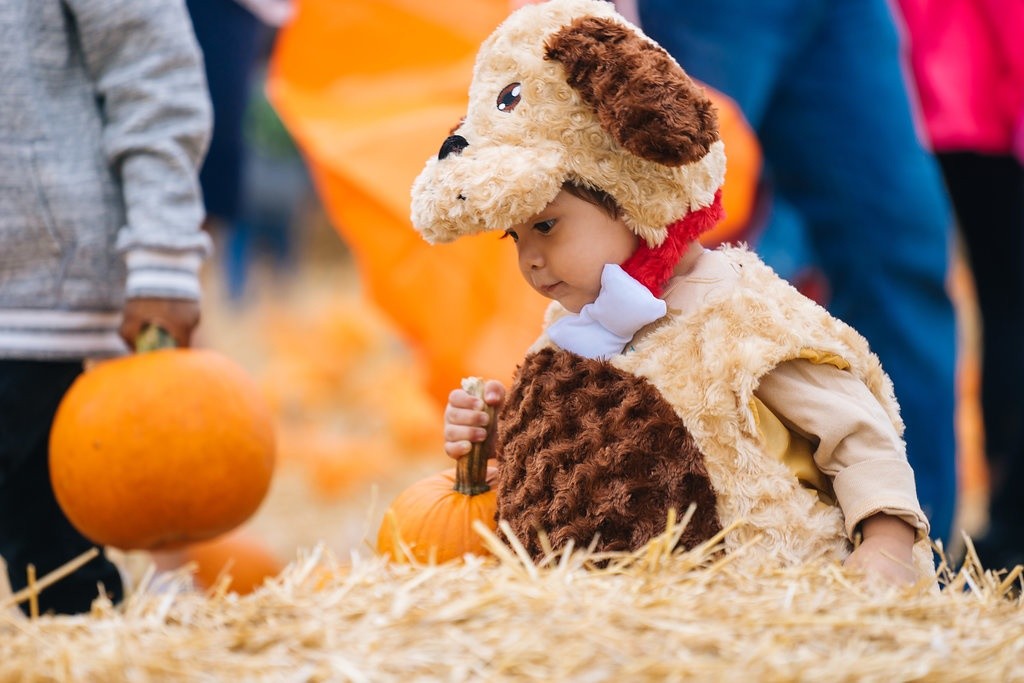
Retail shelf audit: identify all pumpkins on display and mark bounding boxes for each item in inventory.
[377,376,501,569]
[50,325,288,593]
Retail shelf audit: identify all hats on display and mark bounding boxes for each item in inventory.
[409,0,727,360]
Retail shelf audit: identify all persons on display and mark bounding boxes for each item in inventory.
[411,0,941,599]
[0,0,215,619]
[632,0,961,592]
[179,0,309,304]
[889,0,1024,596]
